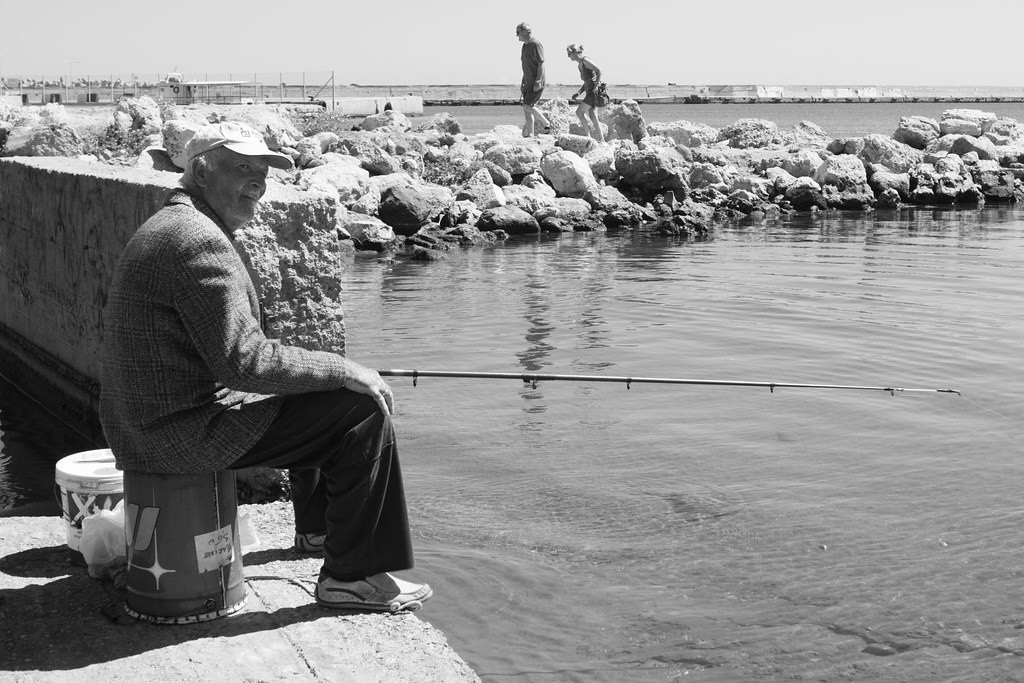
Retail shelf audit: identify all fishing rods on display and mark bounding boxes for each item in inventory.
[379,368,962,397]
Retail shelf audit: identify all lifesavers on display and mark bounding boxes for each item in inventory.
[173,87,179,93]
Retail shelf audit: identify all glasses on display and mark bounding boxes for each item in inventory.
[516,30,524,36]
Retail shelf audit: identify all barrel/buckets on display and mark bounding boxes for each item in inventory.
[53,448,124,567]
[122,469,249,625]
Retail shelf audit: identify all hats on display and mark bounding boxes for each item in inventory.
[184,120,294,171]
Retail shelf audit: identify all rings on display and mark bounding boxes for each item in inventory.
[382,387,390,396]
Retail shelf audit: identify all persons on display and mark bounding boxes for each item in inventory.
[100,121,434,612]
[516,22,551,139]
[566,44,606,146]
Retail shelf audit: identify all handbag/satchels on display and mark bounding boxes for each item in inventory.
[593,83,610,106]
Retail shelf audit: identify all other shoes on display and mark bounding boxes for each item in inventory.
[313,567,433,610]
[294,533,329,551]
[543,124,550,134]
